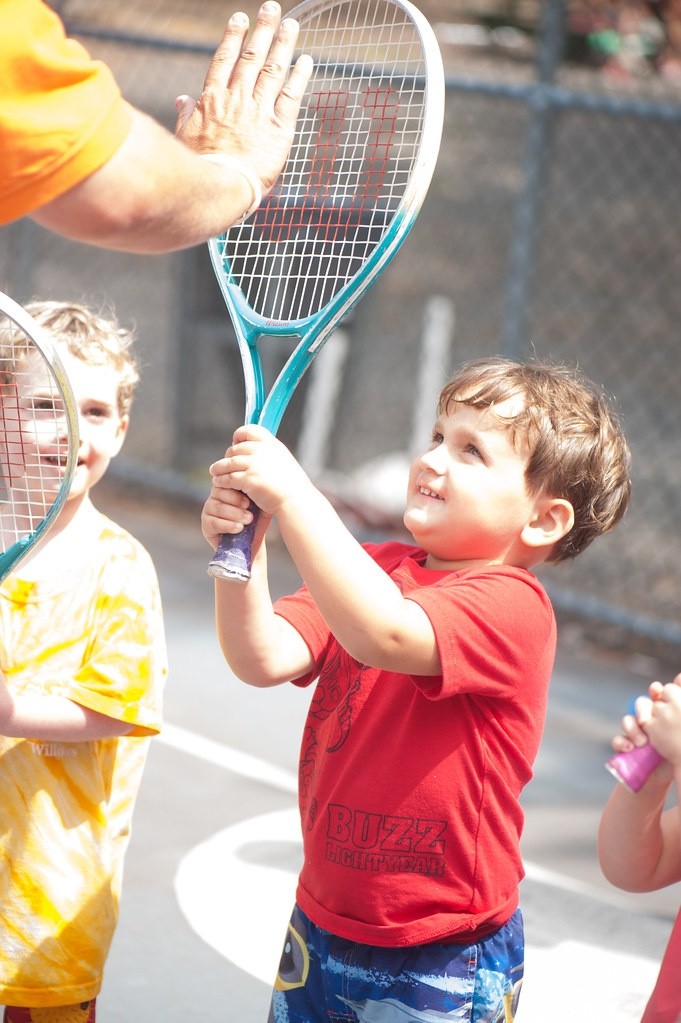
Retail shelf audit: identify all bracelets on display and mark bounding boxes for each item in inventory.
[197,155,260,226]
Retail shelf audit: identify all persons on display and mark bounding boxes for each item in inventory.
[0,300,169,1022]
[598,674,681,1023]
[197,358,631,1023]
[0,0,312,254]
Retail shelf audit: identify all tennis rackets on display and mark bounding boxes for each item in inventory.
[205,2,448,586]
[0,287,82,585]
